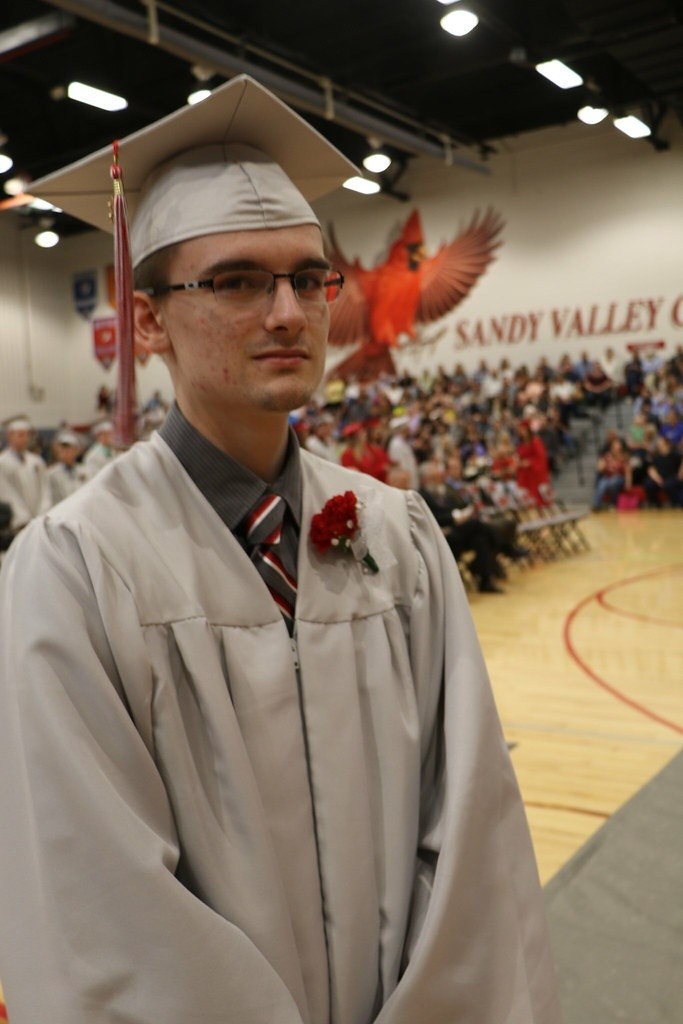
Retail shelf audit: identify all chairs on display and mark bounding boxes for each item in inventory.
[490,484,592,570]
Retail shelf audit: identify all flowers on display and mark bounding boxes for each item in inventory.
[310,492,381,574]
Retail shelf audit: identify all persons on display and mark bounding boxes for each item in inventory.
[0,72,563,1024]
[0,338,683,595]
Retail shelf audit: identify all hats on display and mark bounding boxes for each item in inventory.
[142,399,165,422]
[23,72,361,448]
[54,429,82,447]
[90,418,114,433]
[1,414,32,430]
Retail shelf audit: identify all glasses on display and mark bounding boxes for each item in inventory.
[165,266,345,307]
